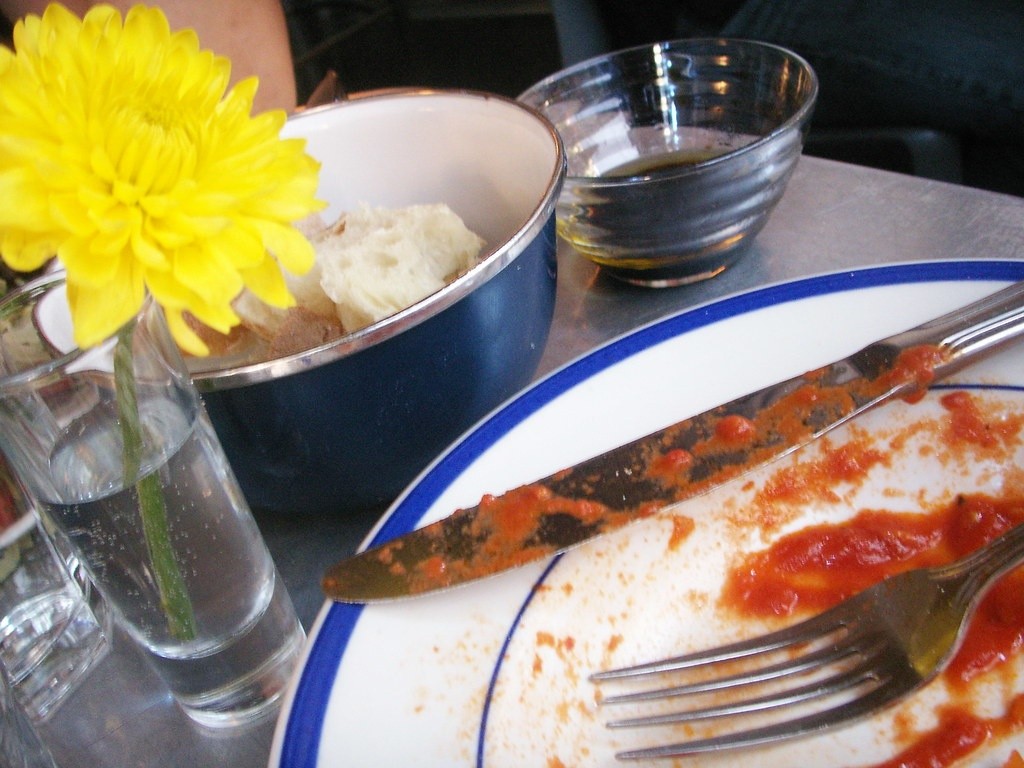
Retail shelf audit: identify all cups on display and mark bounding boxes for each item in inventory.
[0,459,111,722]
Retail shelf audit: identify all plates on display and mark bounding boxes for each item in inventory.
[266,258,1024,768]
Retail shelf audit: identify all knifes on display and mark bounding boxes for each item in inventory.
[319,281,1024,602]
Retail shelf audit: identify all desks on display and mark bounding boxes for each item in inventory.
[0,87,1024,768]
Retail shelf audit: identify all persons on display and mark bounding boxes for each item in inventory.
[0,1,298,270]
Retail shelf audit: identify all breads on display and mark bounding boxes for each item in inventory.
[169,203,483,360]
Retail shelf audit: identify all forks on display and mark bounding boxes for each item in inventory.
[588,524,1024,761]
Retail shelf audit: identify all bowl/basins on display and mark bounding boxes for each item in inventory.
[516,36,819,288]
[30,86,566,530]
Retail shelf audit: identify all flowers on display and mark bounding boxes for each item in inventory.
[0,0,330,645]
